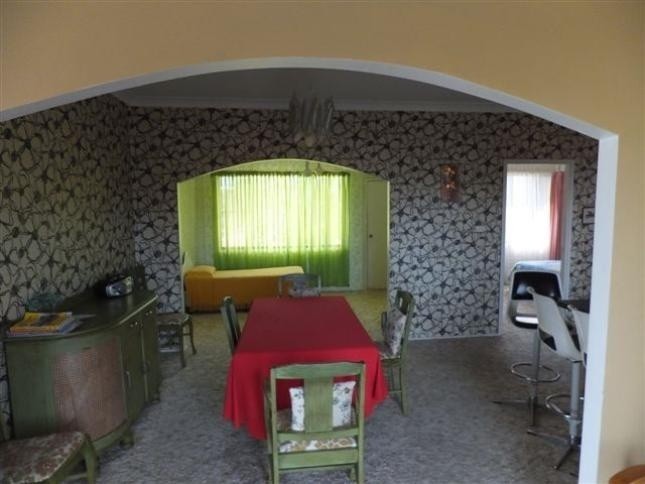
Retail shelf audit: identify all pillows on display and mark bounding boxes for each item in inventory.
[285,376,357,432]
[190,264,218,277]
[380,300,408,357]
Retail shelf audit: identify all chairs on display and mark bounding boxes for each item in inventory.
[275,269,321,299]
[565,300,592,371]
[525,286,585,470]
[495,266,560,431]
[370,287,419,418]
[217,293,241,357]
[130,262,200,369]
[256,360,367,484]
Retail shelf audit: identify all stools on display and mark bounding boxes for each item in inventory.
[1,414,100,483]
[155,309,200,368]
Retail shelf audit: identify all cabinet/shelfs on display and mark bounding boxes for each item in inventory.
[0,271,164,473]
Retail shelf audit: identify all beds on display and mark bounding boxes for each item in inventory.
[507,258,561,282]
[184,264,307,317]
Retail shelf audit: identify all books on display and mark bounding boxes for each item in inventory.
[5,311,81,338]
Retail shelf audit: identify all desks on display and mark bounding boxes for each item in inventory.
[220,291,394,442]
[509,297,590,421]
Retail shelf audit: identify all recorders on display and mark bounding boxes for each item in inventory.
[93,273,134,298]
